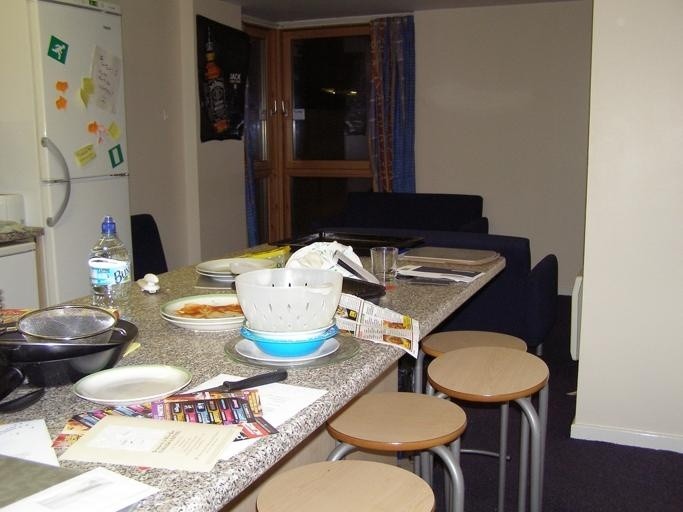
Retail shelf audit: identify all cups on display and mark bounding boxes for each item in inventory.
[370,246,398,282]
[0,193,25,233]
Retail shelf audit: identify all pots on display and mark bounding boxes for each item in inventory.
[0,304,138,403]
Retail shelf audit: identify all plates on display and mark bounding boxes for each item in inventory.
[73,362,194,404]
[225,319,364,372]
[195,258,278,284]
[160,291,245,333]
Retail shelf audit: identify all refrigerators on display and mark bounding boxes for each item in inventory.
[0,0,133,310]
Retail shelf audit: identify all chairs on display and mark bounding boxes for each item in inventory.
[130,212,167,281]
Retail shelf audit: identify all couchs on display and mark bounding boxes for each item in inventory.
[265,228,559,360]
[318,189,488,239]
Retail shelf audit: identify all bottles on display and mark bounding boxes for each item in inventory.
[87,216,139,313]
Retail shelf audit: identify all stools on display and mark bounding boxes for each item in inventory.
[416,332,529,509]
[424,346,549,509]
[254,459,435,510]
[326,390,465,512]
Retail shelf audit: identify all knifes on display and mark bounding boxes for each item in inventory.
[164,368,287,402]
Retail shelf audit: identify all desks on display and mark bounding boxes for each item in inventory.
[0,244,507,512]
